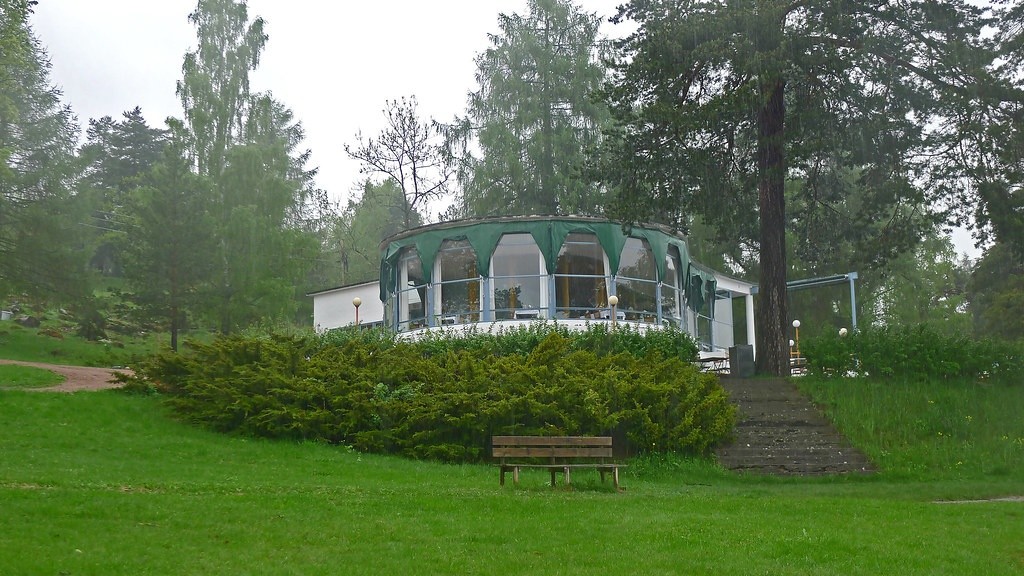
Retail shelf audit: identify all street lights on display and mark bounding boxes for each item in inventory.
[792,320,801,364]
[353,297,362,332]
[606,295,620,337]
[789,340,796,359]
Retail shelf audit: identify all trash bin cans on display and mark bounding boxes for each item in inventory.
[728,344,756,378]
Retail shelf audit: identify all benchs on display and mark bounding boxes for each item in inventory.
[491,433,629,493]
